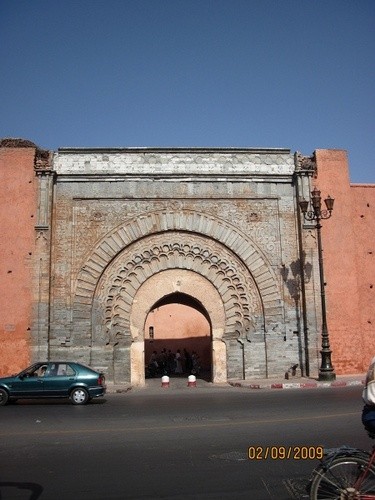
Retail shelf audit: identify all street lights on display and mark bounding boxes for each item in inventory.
[298,186,337,384]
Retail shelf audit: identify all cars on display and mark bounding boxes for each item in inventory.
[1,361,106,405]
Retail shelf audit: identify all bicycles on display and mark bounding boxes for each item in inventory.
[306,427,375,500]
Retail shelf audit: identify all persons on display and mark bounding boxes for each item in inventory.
[358,353,375,443]
[38,366,47,377]
